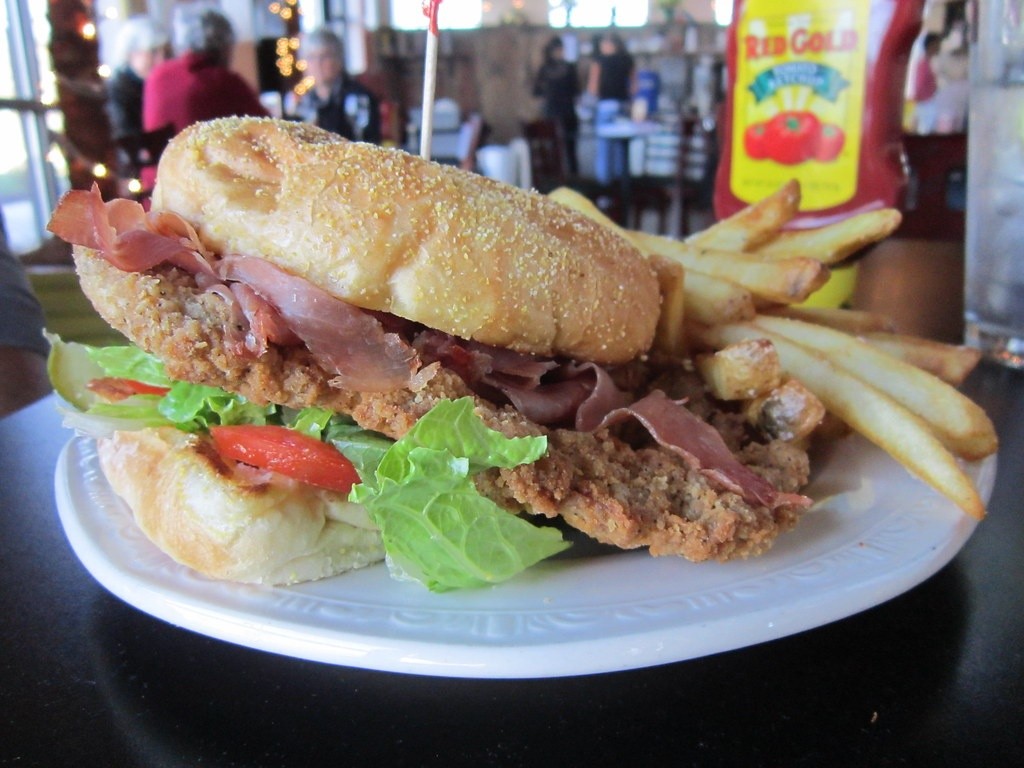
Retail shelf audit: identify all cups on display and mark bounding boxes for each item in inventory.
[962,1,1022,367]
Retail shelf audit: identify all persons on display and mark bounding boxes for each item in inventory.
[0,212,56,420]
[534,38,582,168]
[100,5,272,211]
[916,29,946,137]
[588,31,637,210]
[286,27,382,149]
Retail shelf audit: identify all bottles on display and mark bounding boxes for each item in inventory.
[712,0,924,314]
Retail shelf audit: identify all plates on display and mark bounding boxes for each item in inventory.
[51,432,996,674]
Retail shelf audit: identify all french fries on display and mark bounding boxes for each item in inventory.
[543,178,996,523]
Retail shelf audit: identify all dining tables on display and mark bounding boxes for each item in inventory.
[2,338,1024,767]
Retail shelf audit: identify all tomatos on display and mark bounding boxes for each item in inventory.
[743,109,845,164]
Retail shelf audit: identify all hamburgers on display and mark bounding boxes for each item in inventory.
[43,119,812,592]
